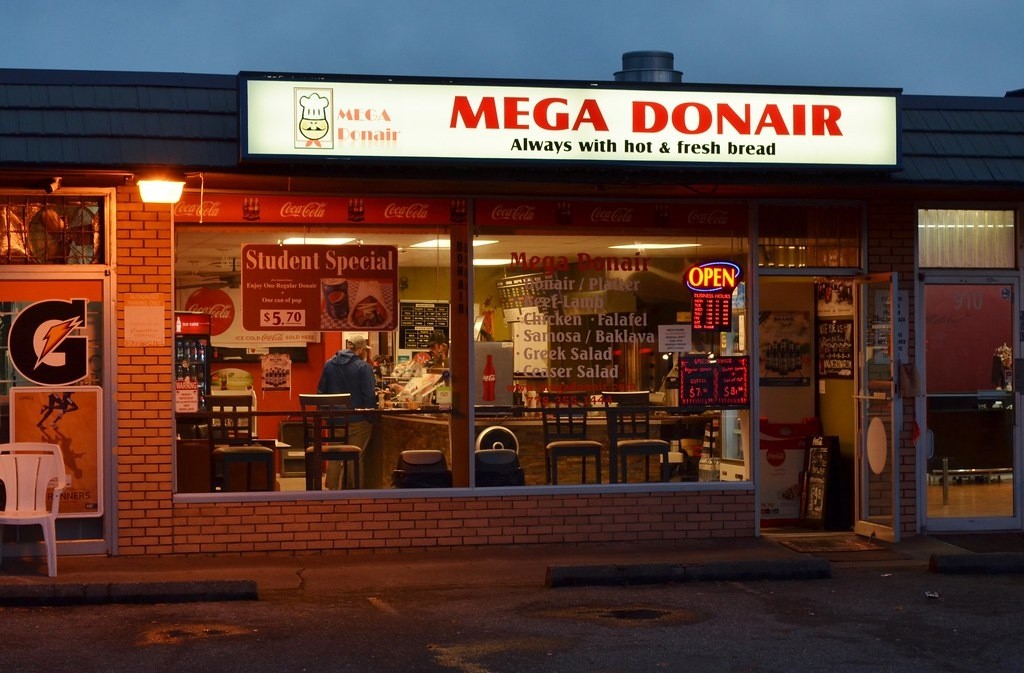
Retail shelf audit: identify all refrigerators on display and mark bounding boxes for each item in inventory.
[722,281,819,518]
[174,311,211,440]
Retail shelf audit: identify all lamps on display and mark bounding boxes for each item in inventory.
[136,172,188,204]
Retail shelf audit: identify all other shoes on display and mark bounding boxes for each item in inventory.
[50,422,58,428]
[40,404,46,414]
[37,423,46,428]
[58,412,63,419]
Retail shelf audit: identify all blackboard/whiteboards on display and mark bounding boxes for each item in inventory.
[801,434,835,522]
[677,354,749,410]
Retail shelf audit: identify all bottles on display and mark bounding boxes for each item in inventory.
[699,457,720,481]
[221,370,227,390]
[451,198,468,221]
[243,196,260,220]
[176,317,182,332]
[512,383,523,416]
[483,354,495,401]
[265,366,289,386]
[177,365,203,384]
[348,198,365,222]
[200,389,205,407]
[178,341,204,361]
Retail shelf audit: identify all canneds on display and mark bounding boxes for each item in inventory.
[321,278,349,320]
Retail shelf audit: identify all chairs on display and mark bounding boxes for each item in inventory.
[299,394,361,491]
[204,394,274,492]
[392,450,453,489]
[539,390,603,485]
[602,390,668,485]
[475,449,524,487]
[0,442,66,577]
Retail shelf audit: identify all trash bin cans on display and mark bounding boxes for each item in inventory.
[475,449,525,488]
[393,448,447,489]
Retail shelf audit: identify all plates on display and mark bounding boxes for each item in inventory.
[348,281,392,328]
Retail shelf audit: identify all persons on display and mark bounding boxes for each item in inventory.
[809,485,822,507]
[371,354,392,379]
[819,279,853,304]
[36,392,79,430]
[811,451,822,473]
[317,335,379,491]
[987,381,1013,409]
[423,332,450,368]
[672,330,711,482]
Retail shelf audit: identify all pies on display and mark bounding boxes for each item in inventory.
[347,282,393,329]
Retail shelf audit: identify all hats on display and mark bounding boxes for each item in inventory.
[348,334,373,349]
[425,331,447,345]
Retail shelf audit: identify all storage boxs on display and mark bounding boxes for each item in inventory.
[435,387,452,403]
[508,322,549,376]
